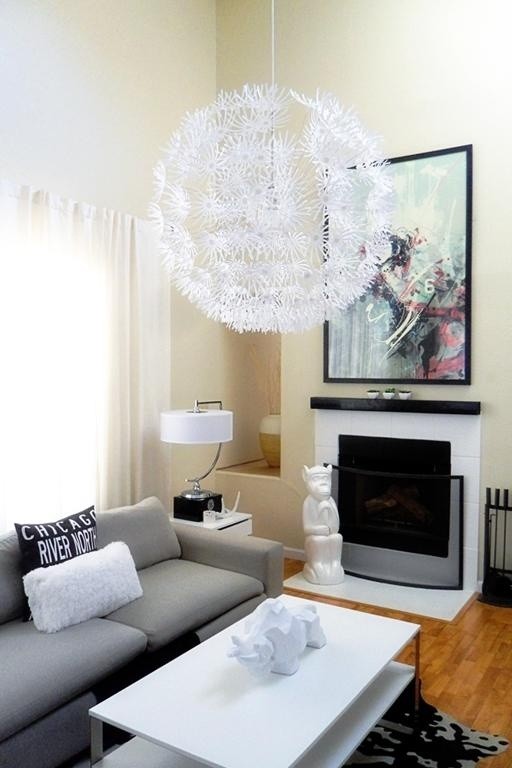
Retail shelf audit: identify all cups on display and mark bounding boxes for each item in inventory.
[203,510,216,524]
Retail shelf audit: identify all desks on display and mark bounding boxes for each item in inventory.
[169,513,255,534]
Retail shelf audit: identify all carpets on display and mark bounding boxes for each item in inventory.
[343,679,511,767]
[283,569,482,626]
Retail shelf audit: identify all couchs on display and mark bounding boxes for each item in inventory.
[1,499,283,768]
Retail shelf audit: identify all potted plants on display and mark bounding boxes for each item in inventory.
[367,389,411,400]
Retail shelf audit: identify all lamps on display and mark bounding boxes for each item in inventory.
[158,398,235,500]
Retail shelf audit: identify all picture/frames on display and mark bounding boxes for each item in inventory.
[324,143,473,387]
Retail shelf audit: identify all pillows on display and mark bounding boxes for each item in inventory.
[14,505,145,633]
